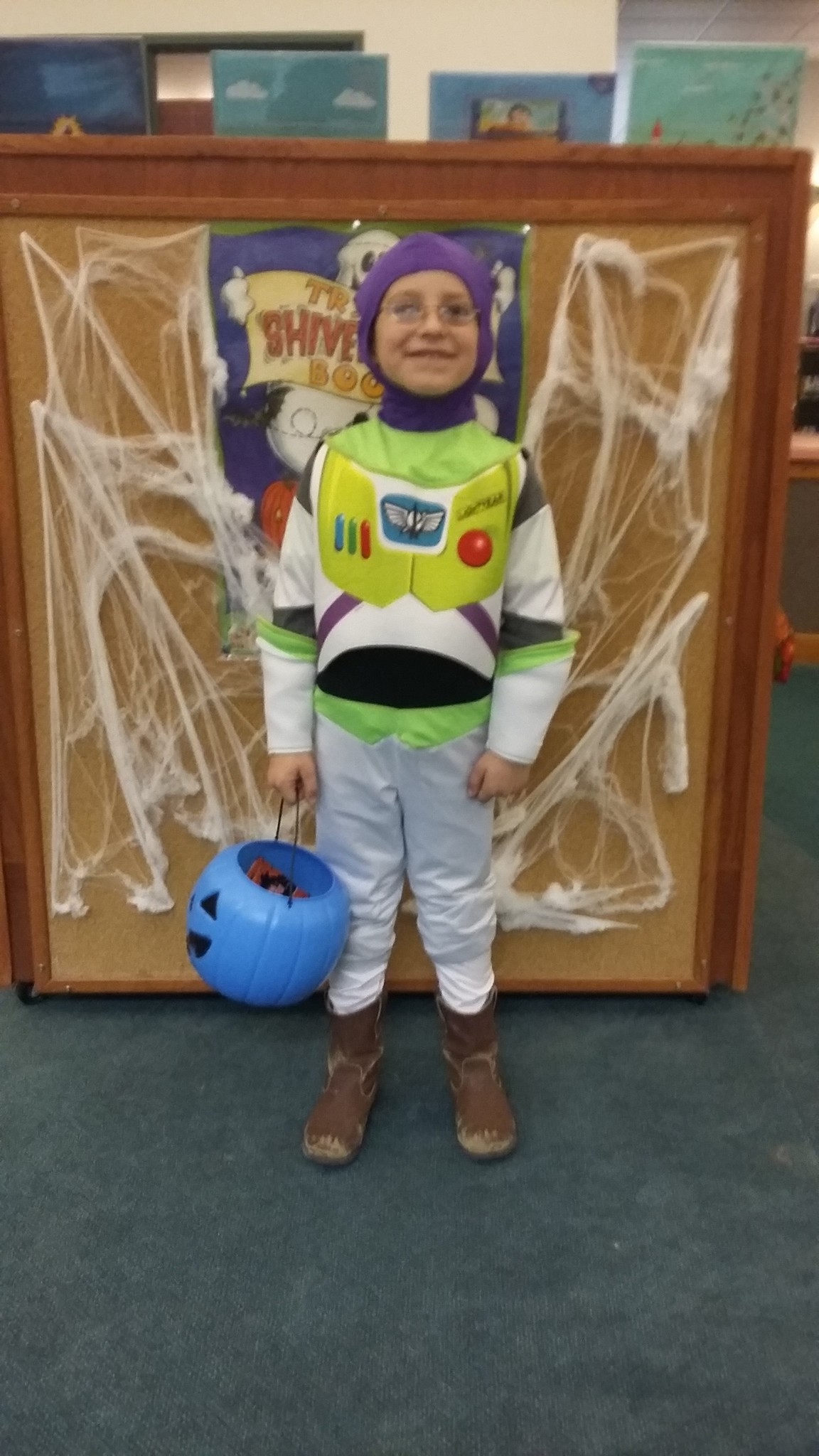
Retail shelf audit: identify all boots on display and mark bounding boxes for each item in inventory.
[435,982,516,1159]
[305,989,384,1164]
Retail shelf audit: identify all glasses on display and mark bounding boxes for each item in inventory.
[377,295,480,326]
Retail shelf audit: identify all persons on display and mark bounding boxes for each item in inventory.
[254,227,570,1165]
